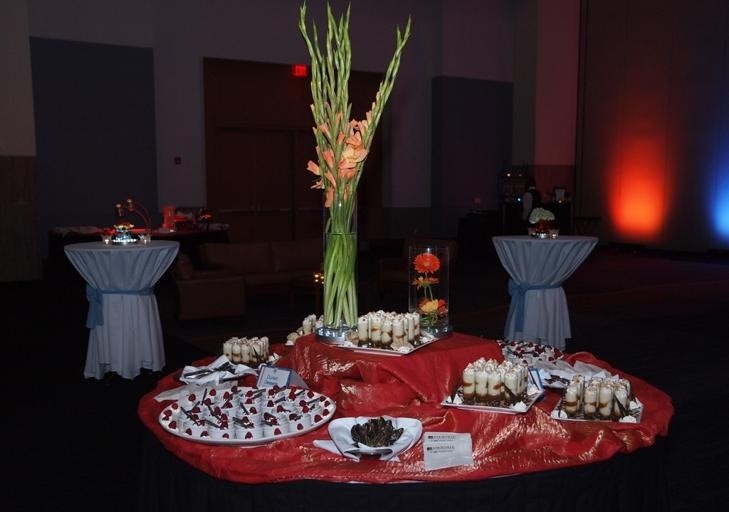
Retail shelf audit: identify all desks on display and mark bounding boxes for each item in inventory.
[491,235,598,352]
[64,240,180,380]
[289,275,322,316]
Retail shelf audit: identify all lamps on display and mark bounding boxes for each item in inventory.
[116,195,153,238]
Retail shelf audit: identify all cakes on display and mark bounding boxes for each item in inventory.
[162,384,333,441]
[498,339,563,367]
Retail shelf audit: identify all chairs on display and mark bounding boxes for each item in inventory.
[171,256,247,320]
[383,240,459,283]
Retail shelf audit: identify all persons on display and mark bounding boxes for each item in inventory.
[518,175,542,235]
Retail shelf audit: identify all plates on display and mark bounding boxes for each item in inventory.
[337,330,440,358]
[159,386,337,443]
[442,381,643,426]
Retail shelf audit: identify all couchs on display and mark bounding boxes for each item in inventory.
[198,241,322,297]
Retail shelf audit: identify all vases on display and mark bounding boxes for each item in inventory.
[323,191,360,332]
[536,221,548,232]
[409,246,451,336]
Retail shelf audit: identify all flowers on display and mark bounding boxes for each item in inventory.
[299,0,413,337]
[413,253,448,329]
[529,207,555,223]
